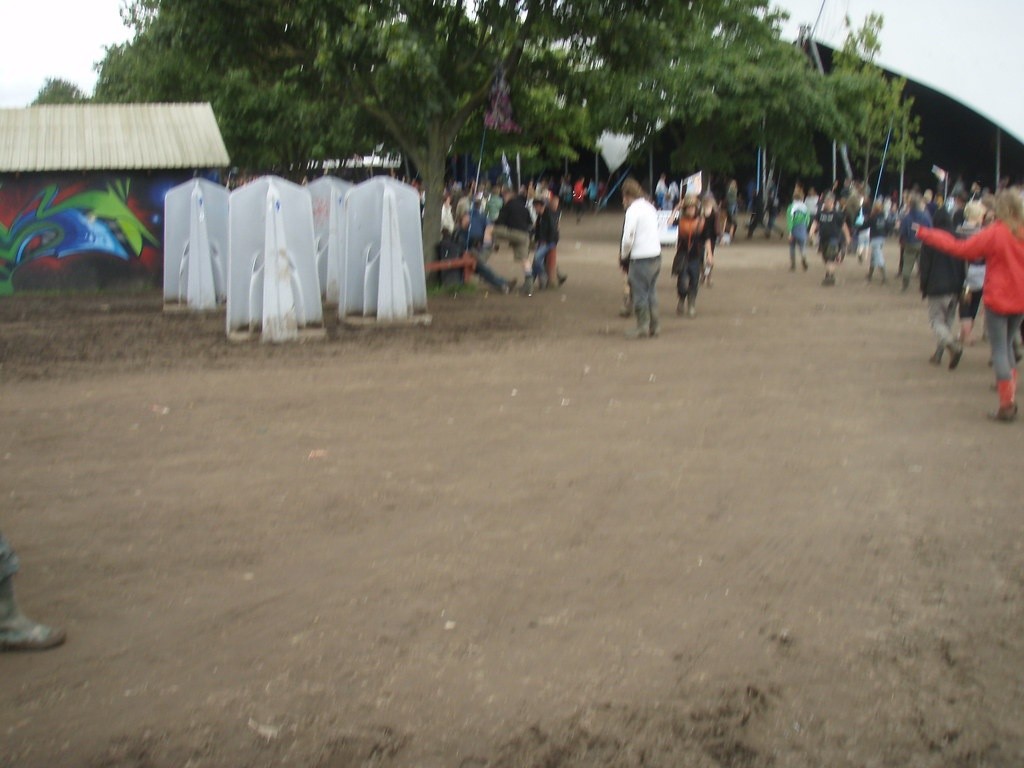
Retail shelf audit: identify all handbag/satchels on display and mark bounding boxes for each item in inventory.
[960,288,971,306]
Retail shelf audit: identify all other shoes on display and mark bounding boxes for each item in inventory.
[928,353,940,364]
[949,344,963,368]
[678,302,684,313]
[687,304,696,316]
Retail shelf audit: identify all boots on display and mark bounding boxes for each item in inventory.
[0,579,65,650]
[1011,369,1017,416]
[998,378,1018,420]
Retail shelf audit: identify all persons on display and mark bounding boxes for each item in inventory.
[0,527,68,653]
[913,184,1024,424]
[409,179,984,368]
